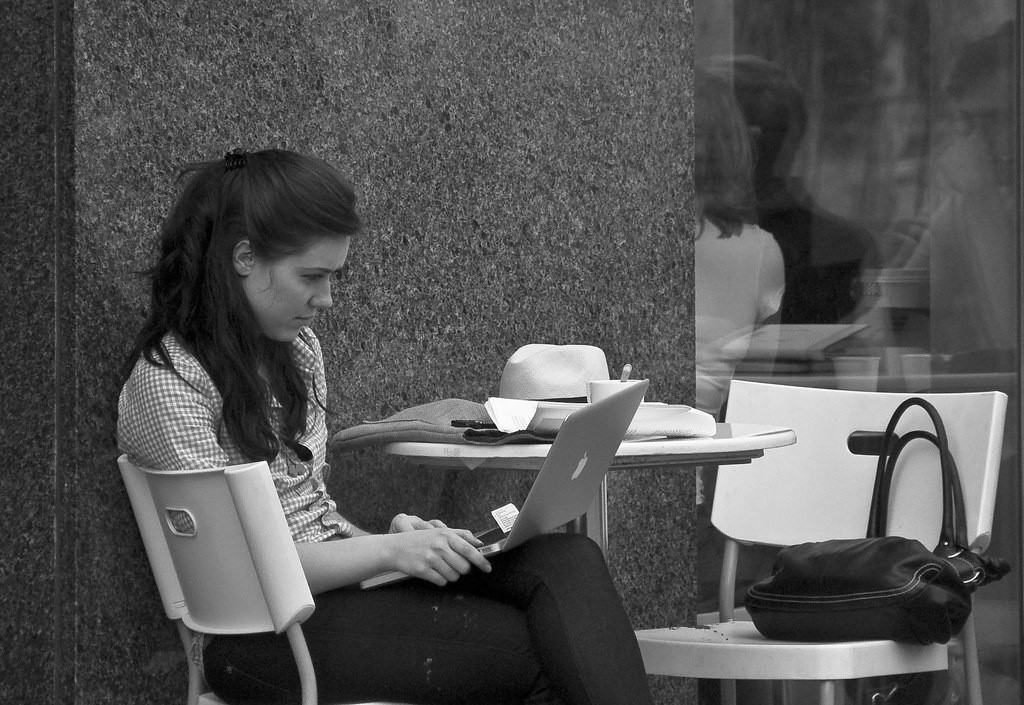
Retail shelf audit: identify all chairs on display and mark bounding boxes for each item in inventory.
[631,378,1010,705]
[117,454,318,705]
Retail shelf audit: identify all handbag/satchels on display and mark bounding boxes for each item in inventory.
[330,398,544,449]
[744,398,1009,646]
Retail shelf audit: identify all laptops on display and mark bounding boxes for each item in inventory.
[361,379,649,588]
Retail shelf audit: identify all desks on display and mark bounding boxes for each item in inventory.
[384,430,797,563]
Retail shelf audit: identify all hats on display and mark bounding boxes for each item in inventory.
[501,343,609,402]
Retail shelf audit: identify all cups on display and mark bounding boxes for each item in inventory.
[590,380,645,404]
[902,355,948,390]
[833,358,879,391]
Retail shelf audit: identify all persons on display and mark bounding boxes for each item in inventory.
[693,66,788,515]
[115,147,654,705]
